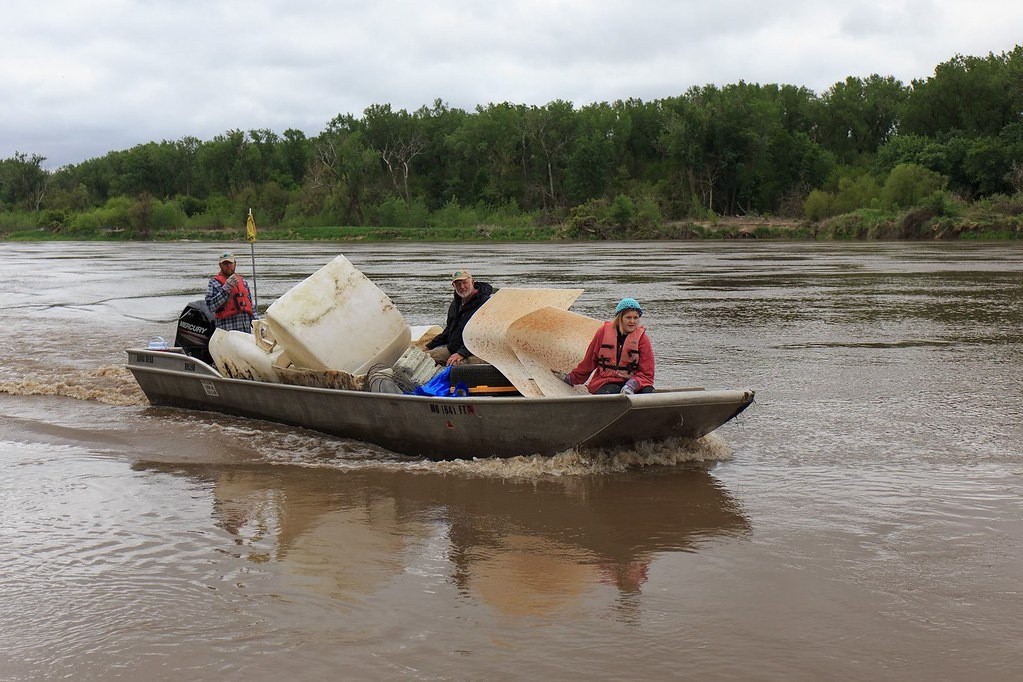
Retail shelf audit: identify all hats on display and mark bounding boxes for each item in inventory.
[615,298,643,318]
[451,270,471,285]
[219,251,235,265]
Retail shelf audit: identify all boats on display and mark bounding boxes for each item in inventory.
[125,326,755,463]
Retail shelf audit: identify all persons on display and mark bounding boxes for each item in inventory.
[206,251,259,333]
[550,298,655,394]
[423,270,492,367]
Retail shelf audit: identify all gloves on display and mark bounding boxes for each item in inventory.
[621,378,638,395]
[222,274,239,289]
[551,368,571,387]
